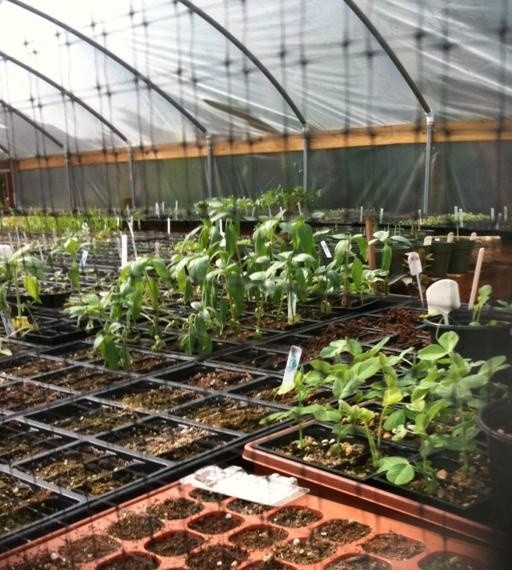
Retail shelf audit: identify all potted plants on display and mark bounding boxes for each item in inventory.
[0,182,512,499]
